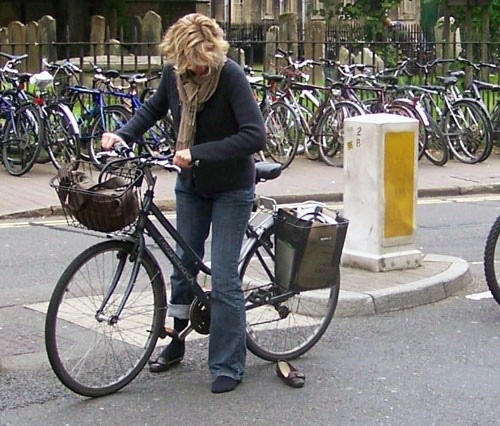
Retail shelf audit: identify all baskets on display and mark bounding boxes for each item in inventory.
[272,207,349,292]
[49,165,144,232]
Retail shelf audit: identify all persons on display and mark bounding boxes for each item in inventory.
[102,13,266,394]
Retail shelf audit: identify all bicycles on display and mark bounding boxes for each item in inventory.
[0,45,500,175]
[44,141,350,397]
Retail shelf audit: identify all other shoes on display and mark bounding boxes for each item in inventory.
[149,353,185,372]
[56,167,138,233]
[276,359,306,388]
